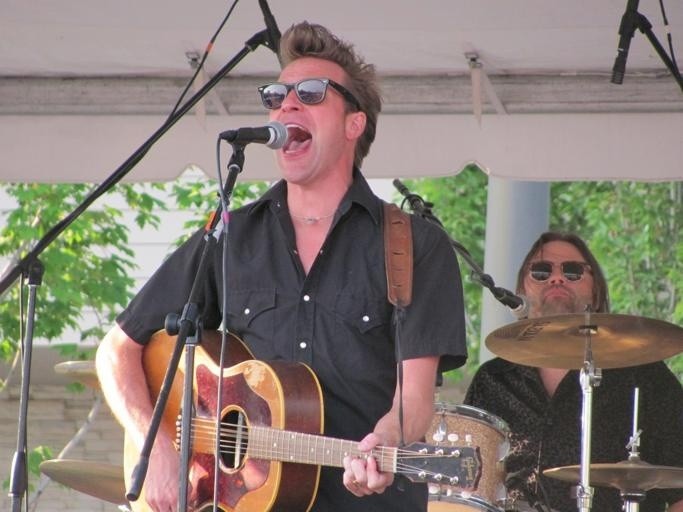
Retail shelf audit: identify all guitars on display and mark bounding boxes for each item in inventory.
[122,328,482,512]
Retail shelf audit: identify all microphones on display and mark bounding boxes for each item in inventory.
[219,120,290,150]
[258,0,282,54]
[494,287,532,318]
[611,0,640,85]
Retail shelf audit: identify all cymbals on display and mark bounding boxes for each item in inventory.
[542,459,682,493]
[39,459,127,507]
[53,361,100,389]
[484,313,683,371]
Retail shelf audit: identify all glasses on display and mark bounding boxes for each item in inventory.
[527,260,590,282]
[258,77,360,110]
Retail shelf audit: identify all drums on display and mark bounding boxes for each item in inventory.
[427,407,514,512]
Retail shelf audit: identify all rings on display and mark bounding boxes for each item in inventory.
[351,480,360,490]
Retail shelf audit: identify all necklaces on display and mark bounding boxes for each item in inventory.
[286,208,336,227]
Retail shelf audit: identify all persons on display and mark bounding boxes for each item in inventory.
[89,18,468,511]
[453,231,681,512]
